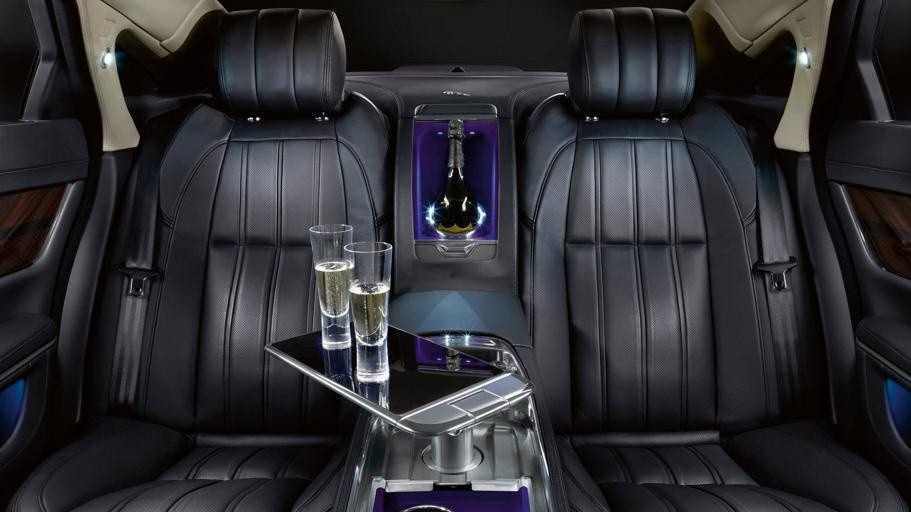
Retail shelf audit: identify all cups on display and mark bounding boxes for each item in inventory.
[309,223,356,350]
[344,240,393,383]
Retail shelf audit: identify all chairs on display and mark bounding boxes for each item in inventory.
[10,7,394,510]
[519,8,908,511]
